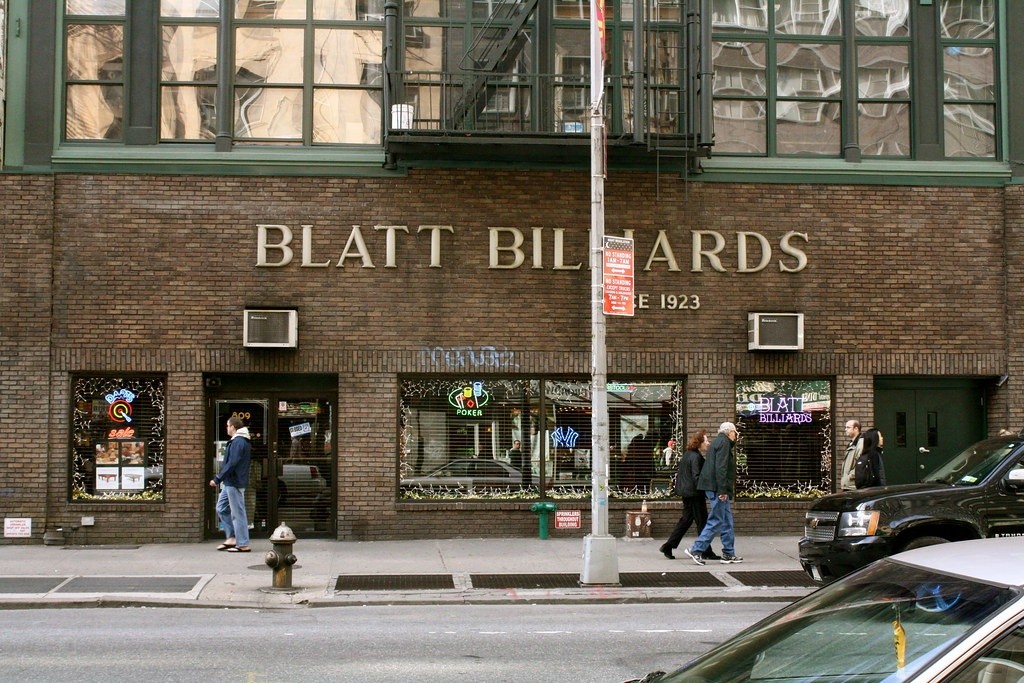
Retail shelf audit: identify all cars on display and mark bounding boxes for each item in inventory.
[623,536,1024,683]
[399,457,555,498]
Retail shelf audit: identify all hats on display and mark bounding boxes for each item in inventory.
[668,440,676,446]
[720,422,738,434]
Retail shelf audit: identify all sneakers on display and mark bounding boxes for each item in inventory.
[685,548,705,565]
[720,554,743,564]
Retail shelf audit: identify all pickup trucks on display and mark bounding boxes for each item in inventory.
[215,440,327,508]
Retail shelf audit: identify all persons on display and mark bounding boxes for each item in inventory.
[660,441,676,468]
[576,449,589,466]
[290,435,300,458]
[660,430,721,560]
[841,420,864,491]
[685,422,743,566]
[862,428,886,489]
[210,417,253,553]
[509,440,521,471]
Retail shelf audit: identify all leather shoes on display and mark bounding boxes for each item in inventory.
[659,544,675,559]
[701,550,721,559]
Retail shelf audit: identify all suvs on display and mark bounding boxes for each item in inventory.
[798,433,1024,625]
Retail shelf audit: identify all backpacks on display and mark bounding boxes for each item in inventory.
[855,451,877,489]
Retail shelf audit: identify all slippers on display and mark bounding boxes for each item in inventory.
[217,543,236,550]
[228,546,251,552]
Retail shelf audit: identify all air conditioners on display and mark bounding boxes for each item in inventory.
[243,308,297,347]
[746,312,803,350]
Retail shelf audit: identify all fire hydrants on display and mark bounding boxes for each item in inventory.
[530,502,557,540]
[264,520,299,591]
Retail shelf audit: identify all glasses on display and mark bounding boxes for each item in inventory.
[845,425,853,430]
